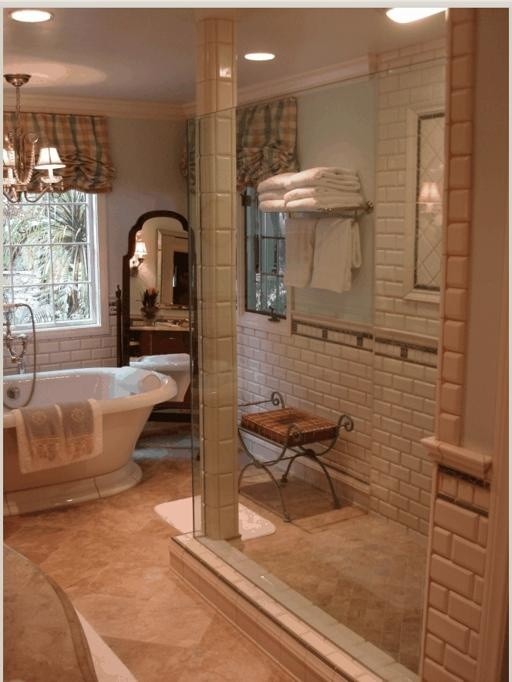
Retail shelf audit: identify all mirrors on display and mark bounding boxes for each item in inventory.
[115,209,195,425]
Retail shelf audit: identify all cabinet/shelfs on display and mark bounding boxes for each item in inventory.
[129,330,193,409]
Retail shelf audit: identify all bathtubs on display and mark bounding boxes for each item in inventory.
[128,353,194,404]
[3,366,179,492]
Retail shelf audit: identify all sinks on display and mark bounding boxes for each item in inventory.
[129,320,177,331]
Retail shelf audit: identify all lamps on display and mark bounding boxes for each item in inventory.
[130,240,148,267]
[413,179,444,227]
[3,71,67,207]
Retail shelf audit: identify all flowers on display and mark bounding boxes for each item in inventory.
[136,287,161,323]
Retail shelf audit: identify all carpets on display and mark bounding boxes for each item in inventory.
[153,490,277,549]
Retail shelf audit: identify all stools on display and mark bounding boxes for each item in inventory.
[237,391,355,522]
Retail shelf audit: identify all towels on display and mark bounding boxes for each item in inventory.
[10,398,105,475]
[257,164,367,296]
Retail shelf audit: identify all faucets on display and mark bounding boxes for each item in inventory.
[166,318,188,327]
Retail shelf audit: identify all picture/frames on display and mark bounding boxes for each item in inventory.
[401,97,445,305]
